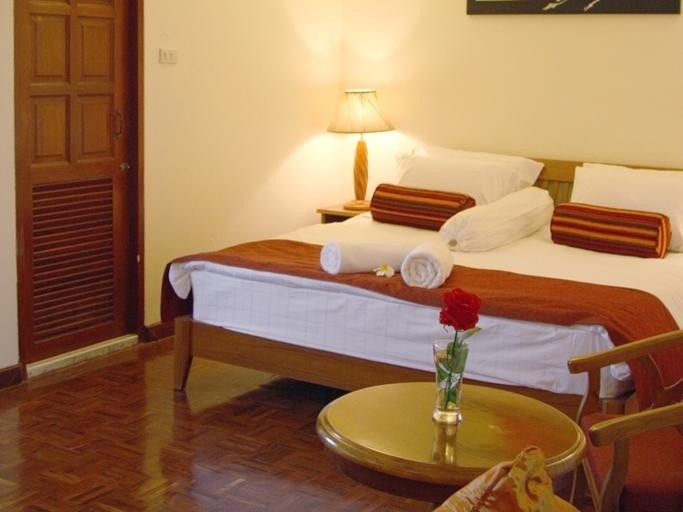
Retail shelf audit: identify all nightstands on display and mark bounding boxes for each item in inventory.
[316,206,365,223]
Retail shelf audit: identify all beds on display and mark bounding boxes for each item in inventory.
[158,156,683,422]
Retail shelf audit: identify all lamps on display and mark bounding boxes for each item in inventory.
[326,89,395,210]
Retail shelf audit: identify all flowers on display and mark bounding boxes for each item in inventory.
[434,287,481,410]
[372,262,395,278]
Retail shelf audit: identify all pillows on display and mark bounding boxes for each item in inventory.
[438,186,554,252]
[370,184,475,230]
[570,163,683,252]
[549,203,671,258]
[399,145,545,207]
[431,444,579,512]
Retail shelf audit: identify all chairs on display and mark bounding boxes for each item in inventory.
[568,329,683,512]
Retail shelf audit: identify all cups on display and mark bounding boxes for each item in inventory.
[431,426,457,467]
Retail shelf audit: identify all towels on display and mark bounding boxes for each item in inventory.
[400,242,454,289]
[319,239,411,275]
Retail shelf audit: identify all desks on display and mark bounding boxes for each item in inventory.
[315,381,588,512]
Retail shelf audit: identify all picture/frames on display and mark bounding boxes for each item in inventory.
[467,0,680,15]
